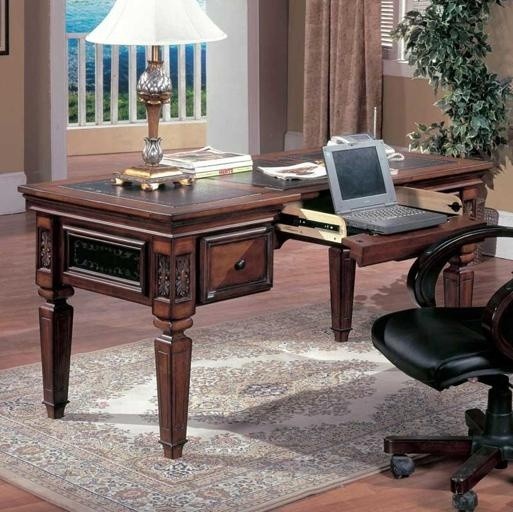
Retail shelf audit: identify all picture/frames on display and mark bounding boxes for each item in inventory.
[0,0,9,56]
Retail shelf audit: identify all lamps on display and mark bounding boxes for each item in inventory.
[84,0,227,192]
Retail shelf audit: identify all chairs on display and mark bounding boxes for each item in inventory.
[369,223,512,510]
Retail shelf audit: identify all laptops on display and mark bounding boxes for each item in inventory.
[322,139,448,235]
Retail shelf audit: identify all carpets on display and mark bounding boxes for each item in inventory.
[0,303,509,511]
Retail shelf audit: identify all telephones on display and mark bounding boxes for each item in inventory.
[327,133,395,156]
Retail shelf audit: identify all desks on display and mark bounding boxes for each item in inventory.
[16,144,494,460]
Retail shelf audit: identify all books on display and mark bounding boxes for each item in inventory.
[183,165,252,178]
[163,146,250,170]
[256,162,327,181]
[313,158,399,176]
[176,160,254,173]
